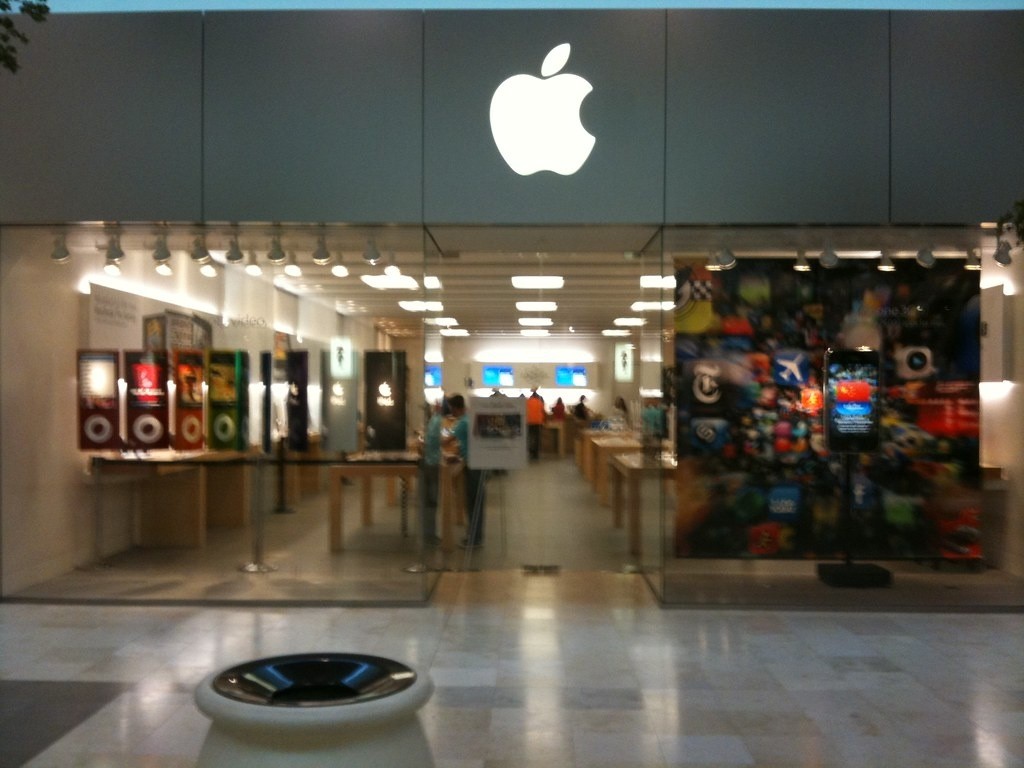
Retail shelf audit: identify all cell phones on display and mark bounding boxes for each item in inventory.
[824,348,881,455]
[75,347,408,456]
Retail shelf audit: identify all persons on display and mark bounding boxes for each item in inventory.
[638,395,670,487]
[552,396,565,444]
[440,393,486,547]
[574,391,628,453]
[421,412,442,545]
[487,383,547,460]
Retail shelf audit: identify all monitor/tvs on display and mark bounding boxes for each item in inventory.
[483,366,514,386]
[556,366,587,387]
[424,366,441,386]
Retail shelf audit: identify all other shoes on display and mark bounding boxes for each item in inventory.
[459,539,482,547]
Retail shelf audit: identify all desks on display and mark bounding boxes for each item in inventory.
[543,413,679,547]
[332,450,463,556]
[89,433,325,551]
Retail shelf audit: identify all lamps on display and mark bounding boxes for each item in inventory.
[704,218,1013,272]
[52,224,381,267]
